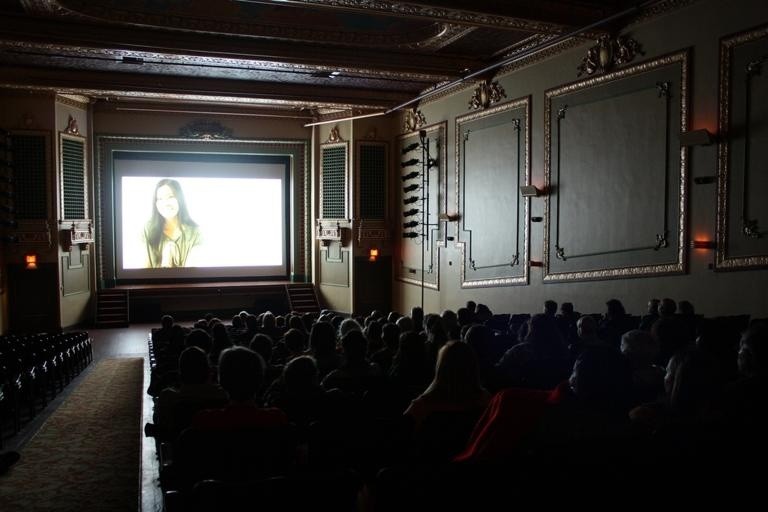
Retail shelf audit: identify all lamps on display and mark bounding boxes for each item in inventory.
[399,129,439,241]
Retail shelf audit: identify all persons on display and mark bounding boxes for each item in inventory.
[151,299,768,512]
[139,179,202,268]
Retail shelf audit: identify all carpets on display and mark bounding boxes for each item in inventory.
[0,356,144,512]
